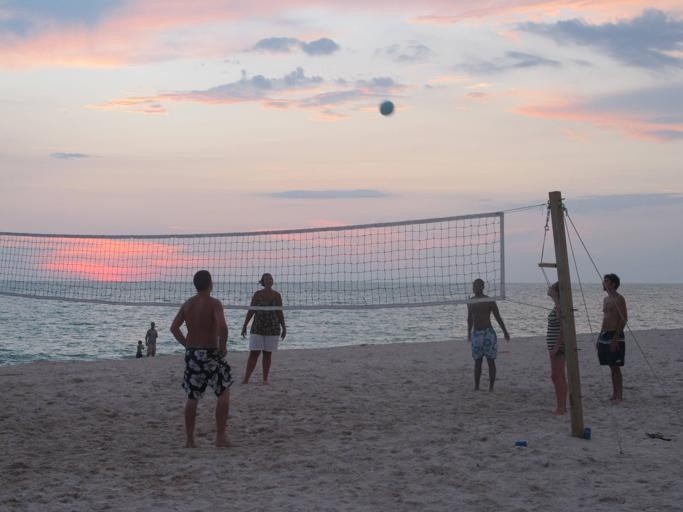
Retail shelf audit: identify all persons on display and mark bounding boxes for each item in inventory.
[171,270,241,448]
[546,281,568,415]
[241,273,286,385]
[467,279,510,392]
[600,274,627,404]
[145,322,158,357]
[136,340,144,358]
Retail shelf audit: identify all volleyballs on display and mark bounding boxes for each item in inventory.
[380,100,393,115]
